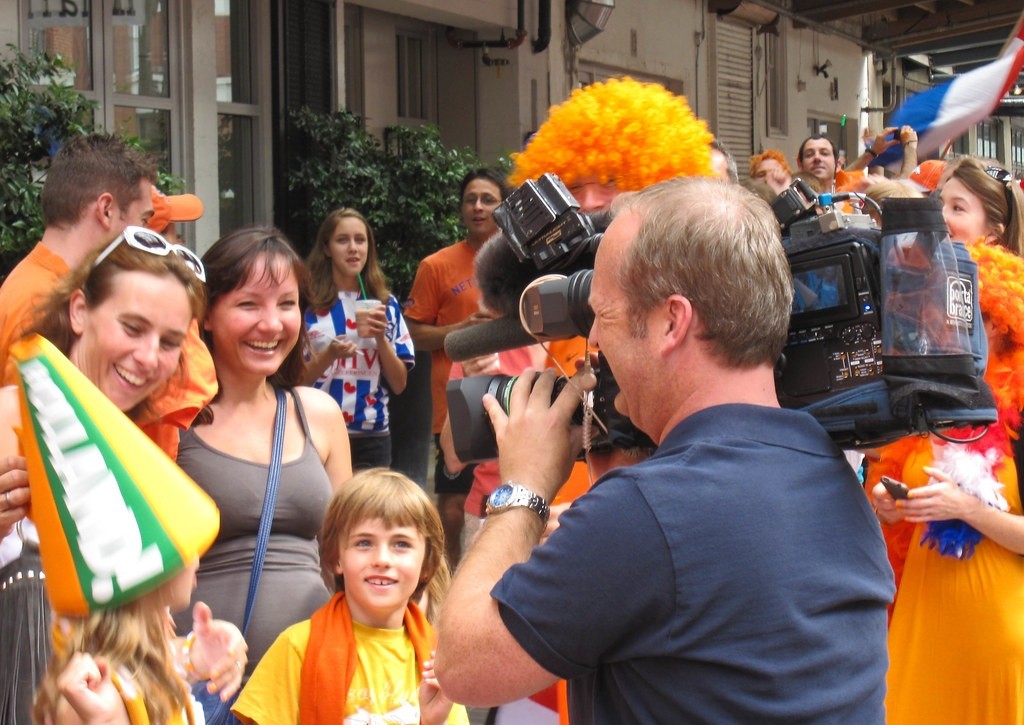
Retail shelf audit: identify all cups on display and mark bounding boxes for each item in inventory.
[352,299,383,339]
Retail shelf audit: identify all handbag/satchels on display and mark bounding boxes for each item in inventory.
[193,680,242,725]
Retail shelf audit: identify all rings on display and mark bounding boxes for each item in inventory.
[4,491,14,509]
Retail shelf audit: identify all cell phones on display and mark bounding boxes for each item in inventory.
[879,475,910,500]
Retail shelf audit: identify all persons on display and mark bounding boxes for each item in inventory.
[0,75,1024,725]
[797,265,839,313]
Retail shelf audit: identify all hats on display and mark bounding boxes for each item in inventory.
[142,185,204,233]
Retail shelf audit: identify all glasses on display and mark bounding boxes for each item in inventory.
[465,195,501,206]
[93,225,207,283]
[985,166,1012,230]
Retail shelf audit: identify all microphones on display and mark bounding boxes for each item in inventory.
[475,234,542,310]
[445,314,540,362]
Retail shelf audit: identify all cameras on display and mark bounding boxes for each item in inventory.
[893,129,900,140]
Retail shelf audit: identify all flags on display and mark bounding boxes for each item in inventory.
[866,16,1024,172]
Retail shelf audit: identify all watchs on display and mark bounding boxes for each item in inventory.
[486,480,551,531]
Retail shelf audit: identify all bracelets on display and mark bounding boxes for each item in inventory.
[865,148,878,158]
